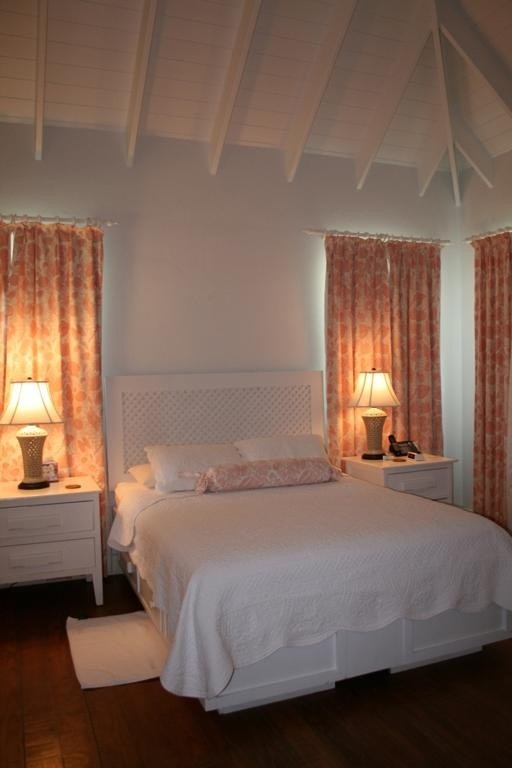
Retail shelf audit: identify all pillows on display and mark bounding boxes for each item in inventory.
[129,433,342,493]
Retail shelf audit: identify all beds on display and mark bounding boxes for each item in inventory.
[103,477,512,719]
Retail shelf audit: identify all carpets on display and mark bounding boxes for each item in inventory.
[64,609,173,691]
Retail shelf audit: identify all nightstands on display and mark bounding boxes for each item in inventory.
[343,452,459,505]
[0,474,104,606]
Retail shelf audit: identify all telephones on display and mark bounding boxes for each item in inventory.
[389,435,421,456]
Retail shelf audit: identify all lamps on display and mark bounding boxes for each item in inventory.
[347,367,401,460]
[0,377,64,489]
